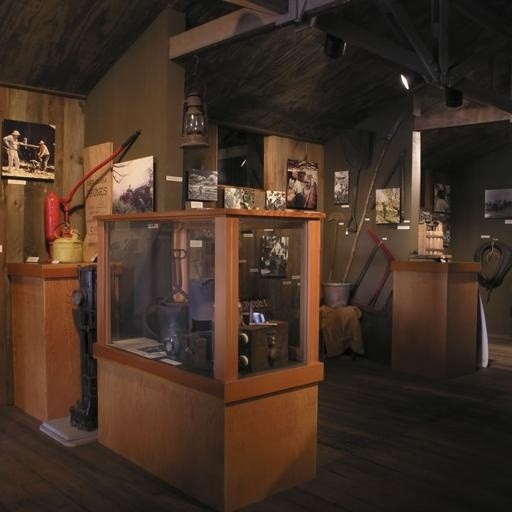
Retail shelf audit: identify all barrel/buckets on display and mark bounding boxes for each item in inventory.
[322,283,352,307]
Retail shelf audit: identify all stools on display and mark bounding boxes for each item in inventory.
[319,304,364,364]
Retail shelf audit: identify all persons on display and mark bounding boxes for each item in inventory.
[3,130,22,169]
[36,139,50,172]
[436,188,450,217]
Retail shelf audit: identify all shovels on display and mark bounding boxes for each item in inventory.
[343,129,370,233]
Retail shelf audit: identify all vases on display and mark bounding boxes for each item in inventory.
[321,282,356,309]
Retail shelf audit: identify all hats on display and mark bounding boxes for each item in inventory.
[39,140,44,144]
[12,131,20,136]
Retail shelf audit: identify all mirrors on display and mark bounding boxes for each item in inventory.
[216,125,270,193]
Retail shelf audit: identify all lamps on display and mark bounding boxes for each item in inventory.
[323,33,348,60]
[399,67,427,94]
[234,156,249,169]
[446,83,464,113]
[178,51,210,152]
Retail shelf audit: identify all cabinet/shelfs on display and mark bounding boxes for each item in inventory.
[4,259,125,422]
[90,204,329,512]
[389,260,482,380]
